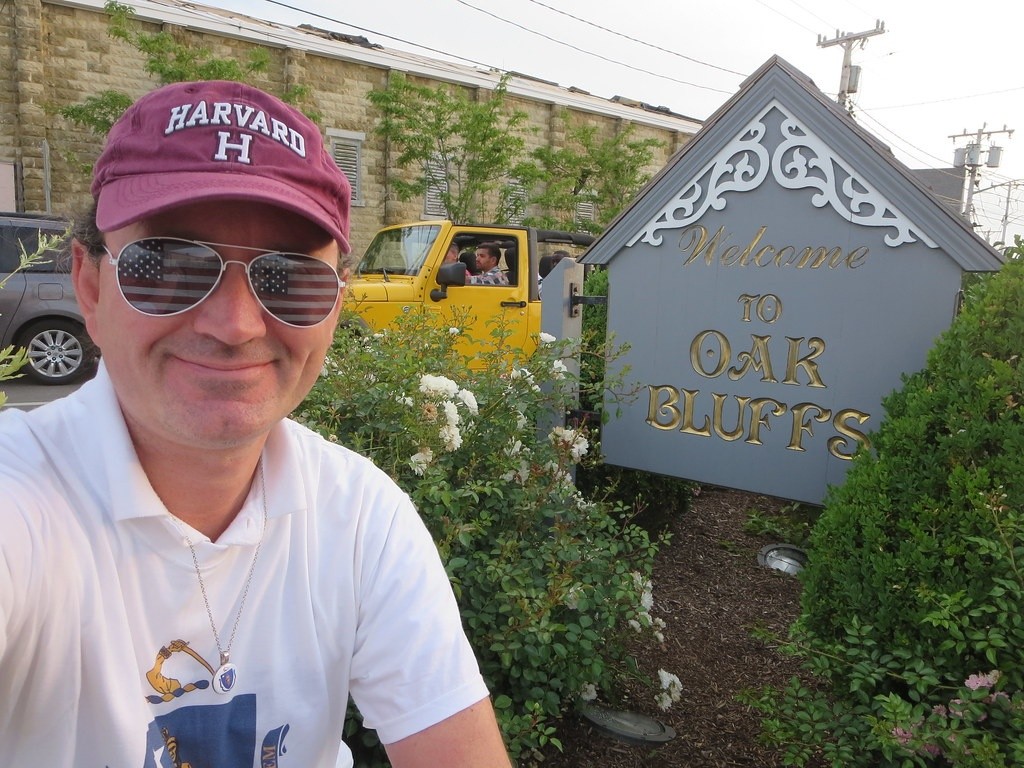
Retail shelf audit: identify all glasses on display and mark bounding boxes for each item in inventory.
[552,259,561,263]
[100,237,345,328]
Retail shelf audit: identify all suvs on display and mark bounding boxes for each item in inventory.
[1,211,97,387]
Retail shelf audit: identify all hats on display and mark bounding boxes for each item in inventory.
[90,81,351,256]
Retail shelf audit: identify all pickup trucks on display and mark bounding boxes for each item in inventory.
[337,219,597,387]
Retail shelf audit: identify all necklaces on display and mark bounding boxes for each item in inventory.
[164,453,268,694]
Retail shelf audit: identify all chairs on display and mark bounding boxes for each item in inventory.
[460,253,476,277]
[504,248,516,285]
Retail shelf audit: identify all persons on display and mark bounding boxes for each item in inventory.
[441,241,472,278]
[0,79,518,768]
[465,243,509,287]
